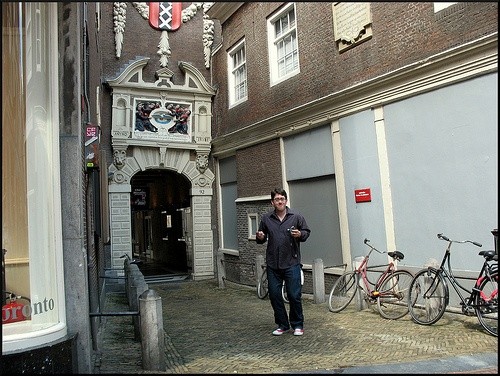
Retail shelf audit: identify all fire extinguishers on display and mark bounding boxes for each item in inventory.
[1,289,31,324]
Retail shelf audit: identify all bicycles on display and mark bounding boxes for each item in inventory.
[408,232,498,337]
[257,259,304,303]
[329,238,420,319]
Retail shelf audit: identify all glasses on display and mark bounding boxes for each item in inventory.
[273,198,287,202]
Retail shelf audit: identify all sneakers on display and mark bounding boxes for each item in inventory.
[272,328,290,336]
[294,328,305,336]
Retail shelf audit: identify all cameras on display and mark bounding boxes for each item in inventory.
[287,226,295,232]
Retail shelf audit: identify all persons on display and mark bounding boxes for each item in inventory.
[256,187,311,336]
